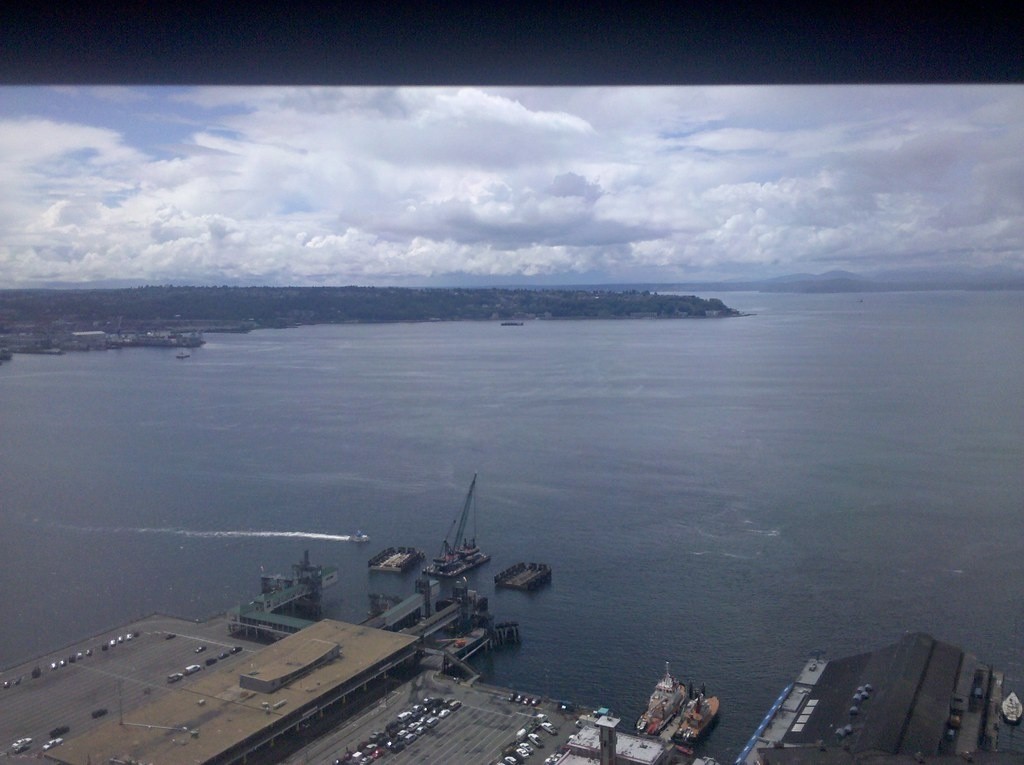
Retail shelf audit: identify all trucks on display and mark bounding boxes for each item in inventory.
[11,708,110,753]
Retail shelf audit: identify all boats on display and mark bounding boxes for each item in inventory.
[350,529,369,542]
[633,661,688,735]
[1002,689,1023,724]
[671,693,720,748]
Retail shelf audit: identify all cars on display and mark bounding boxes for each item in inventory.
[508,692,518,701]
[494,713,580,765]
[3,630,140,690]
[332,693,463,765]
[207,658,215,666]
[230,646,243,654]
[523,695,533,704]
[219,650,229,658]
[166,633,175,640]
[196,646,205,654]
[532,697,540,706]
[515,694,525,702]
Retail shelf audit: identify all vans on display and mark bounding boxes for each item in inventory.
[168,672,183,683]
[185,665,200,674]
